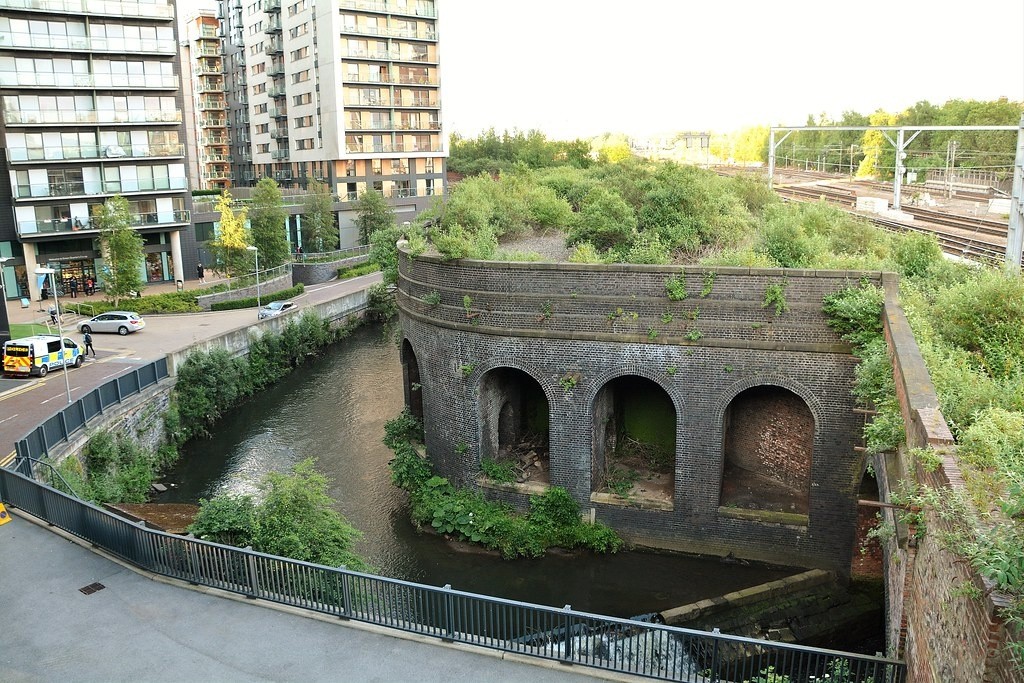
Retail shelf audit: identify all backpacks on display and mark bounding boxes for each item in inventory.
[84,333,92,343]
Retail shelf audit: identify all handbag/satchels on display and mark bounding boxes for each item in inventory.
[51,310,56,315]
[72,282,76,287]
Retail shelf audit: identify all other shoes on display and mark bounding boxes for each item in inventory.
[53,321,55,325]
[93,354,97,358]
[88,296,90,297]
[84,293,86,296]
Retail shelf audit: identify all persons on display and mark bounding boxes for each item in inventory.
[295,245,305,261]
[83,331,97,357]
[197,263,206,283]
[47,302,64,325]
[82,273,95,296]
[69,276,78,298]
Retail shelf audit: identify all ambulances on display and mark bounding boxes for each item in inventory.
[4,334,86,378]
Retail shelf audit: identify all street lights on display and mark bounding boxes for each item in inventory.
[246,246,261,321]
[34,267,73,405]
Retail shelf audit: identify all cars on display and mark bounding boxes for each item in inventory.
[258,300,298,320]
[77,310,145,336]
